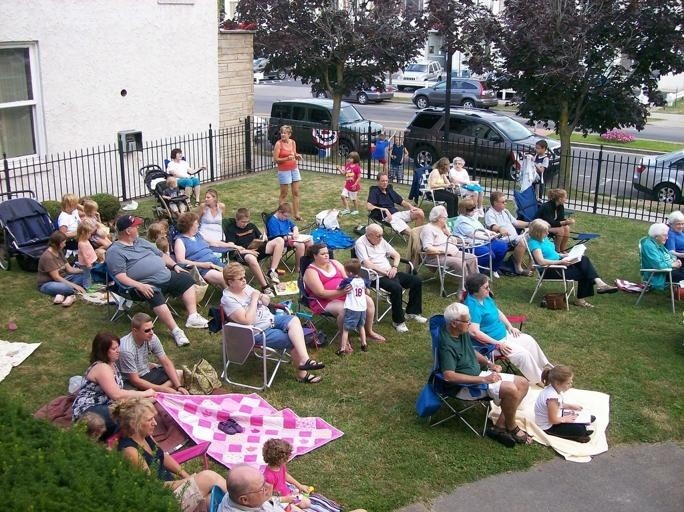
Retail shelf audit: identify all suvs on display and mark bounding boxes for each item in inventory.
[267,96,385,159]
[410,76,500,114]
[395,56,444,95]
[393,101,565,181]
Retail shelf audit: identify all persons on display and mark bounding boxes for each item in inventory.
[437,274,596,445]
[366,130,617,307]
[272,126,303,221]
[71,311,312,511]
[641,210,684,291]
[35,151,428,382]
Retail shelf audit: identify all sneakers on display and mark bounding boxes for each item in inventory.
[341,207,360,216]
[217,417,244,436]
[185,313,211,330]
[334,344,354,357]
[366,333,386,342]
[360,342,370,353]
[170,327,191,348]
[403,312,429,324]
[391,320,410,334]
[294,215,306,222]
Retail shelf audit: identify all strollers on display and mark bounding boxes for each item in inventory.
[0,185,58,273]
[137,165,193,229]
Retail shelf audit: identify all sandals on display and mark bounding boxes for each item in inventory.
[52,293,78,308]
[487,425,534,449]
[597,285,619,295]
[573,300,595,309]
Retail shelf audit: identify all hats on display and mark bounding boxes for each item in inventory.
[116,214,144,232]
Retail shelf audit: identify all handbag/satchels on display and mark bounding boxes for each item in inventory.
[545,292,567,310]
[181,358,224,396]
[415,381,441,419]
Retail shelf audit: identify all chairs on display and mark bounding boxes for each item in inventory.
[636,236,676,313]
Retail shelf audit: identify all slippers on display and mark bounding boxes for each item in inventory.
[297,358,326,372]
[294,371,323,385]
[516,268,534,277]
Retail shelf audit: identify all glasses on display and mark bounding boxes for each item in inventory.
[248,486,267,496]
[455,318,471,325]
[480,284,490,290]
[375,234,385,238]
[497,199,508,205]
[233,275,247,282]
[143,326,155,334]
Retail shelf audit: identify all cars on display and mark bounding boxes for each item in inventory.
[309,72,395,104]
[252,58,294,81]
[633,149,684,204]
